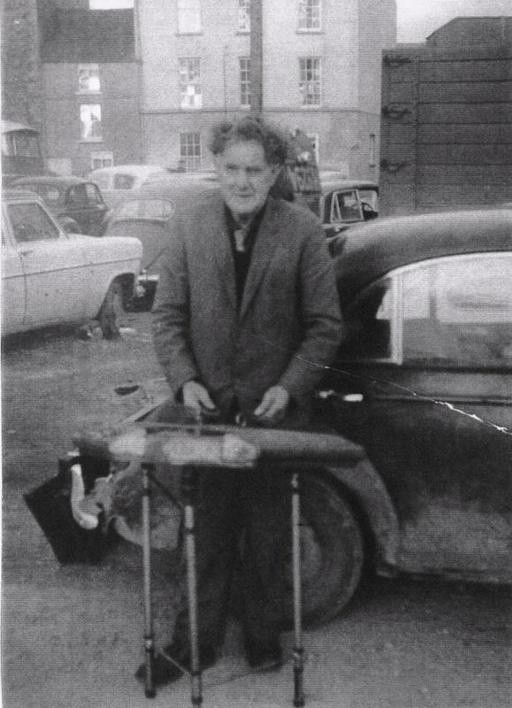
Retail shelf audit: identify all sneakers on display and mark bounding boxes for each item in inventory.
[137,642,215,685]
[244,634,279,671]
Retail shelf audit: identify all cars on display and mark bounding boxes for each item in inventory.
[2,165,220,343]
[299,180,378,246]
[112,210,512,627]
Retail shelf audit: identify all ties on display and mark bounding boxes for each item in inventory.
[234,228,250,254]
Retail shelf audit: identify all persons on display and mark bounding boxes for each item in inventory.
[132,114,345,688]
